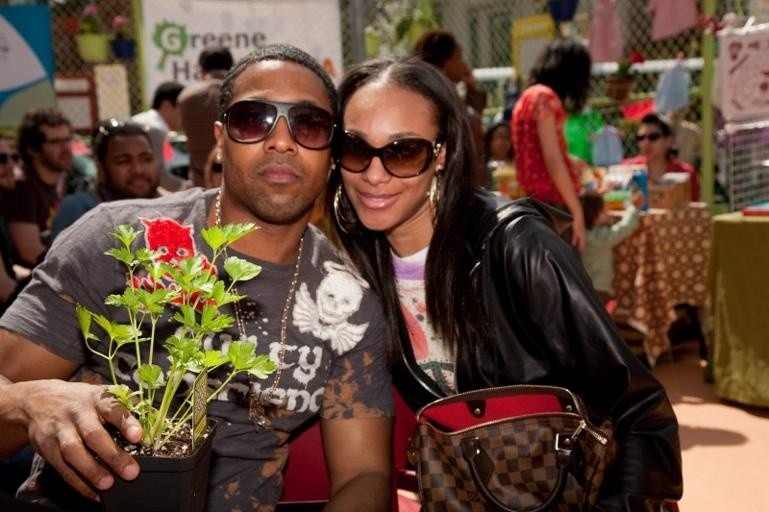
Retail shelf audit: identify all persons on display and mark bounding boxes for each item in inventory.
[512,41,593,253]
[179,48,232,187]
[334,57,683,512]
[129,82,191,195]
[483,123,514,171]
[0,109,72,282]
[204,146,223,188]
[46,118,161,254]
[579,189,642,305]
[620,114,702,202]
[0,144,17,188]
[0,44,393,512]
[0,256,31,303]
[410,32,486,168]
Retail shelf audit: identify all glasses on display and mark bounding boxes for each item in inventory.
[333,130,446,178]
[634,132,662,142]
[94,118,151,143]
[221,98,335,153]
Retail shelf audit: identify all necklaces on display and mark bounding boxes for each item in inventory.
[214,191,305,429]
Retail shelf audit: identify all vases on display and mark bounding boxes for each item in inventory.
[71,32,117,64]
[604,75,637,102]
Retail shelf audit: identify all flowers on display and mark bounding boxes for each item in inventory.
[612,49,647,79]
[64,2,130,45]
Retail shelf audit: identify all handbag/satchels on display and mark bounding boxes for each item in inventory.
[407,385,617,512]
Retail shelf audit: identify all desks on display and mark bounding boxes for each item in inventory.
[566,195,712,368]
[707,200,769,408]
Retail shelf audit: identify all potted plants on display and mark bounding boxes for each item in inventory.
[75,213,279,512]
